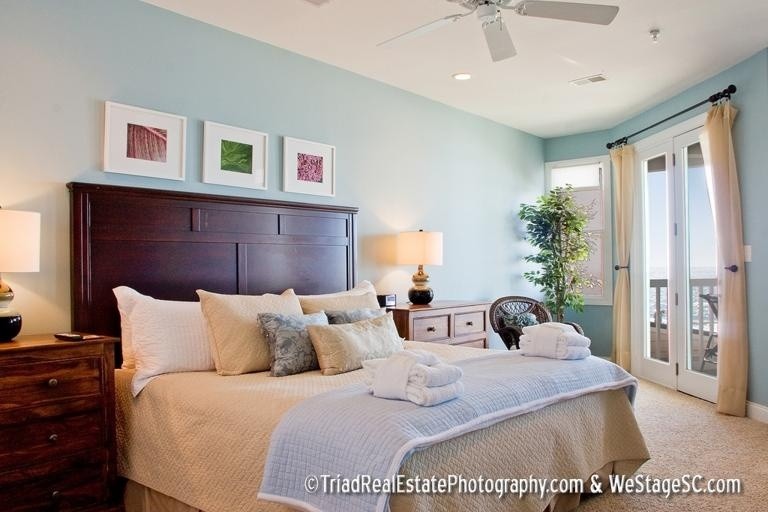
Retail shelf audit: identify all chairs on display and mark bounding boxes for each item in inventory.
[490,295,585,356]
[699,293,720,372]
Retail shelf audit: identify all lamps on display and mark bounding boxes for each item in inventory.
[1,205,41,343]
[399,230,443,304]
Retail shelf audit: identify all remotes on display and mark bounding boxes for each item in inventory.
[54,332,81,342]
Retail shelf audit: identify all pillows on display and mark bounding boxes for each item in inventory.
[256,311,330,378]
[194,289,305,376]
[326,308,386,328]
[297,281,381,315]
[307,310,404,376]
[112,285,217,397]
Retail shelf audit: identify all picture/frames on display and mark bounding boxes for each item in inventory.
[280,135,337,198]
[103,99,187,182]
[201,120,269,191]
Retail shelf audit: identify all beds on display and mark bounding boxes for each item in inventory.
[66,181,651,512]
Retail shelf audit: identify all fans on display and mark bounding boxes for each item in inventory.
[375,2,620,64]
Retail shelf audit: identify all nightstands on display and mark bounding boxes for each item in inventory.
[387,298,493,350]
[0,331,119,512]
